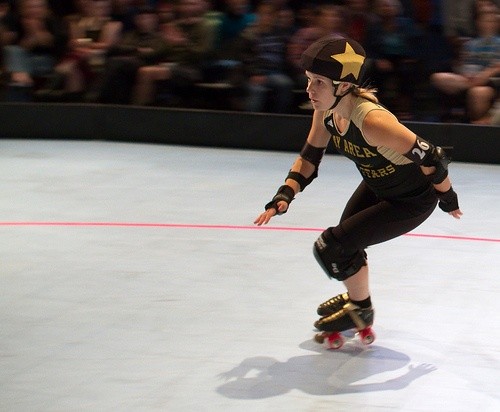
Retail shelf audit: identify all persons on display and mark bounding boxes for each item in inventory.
[1,0,500,128]
[252,34,463,334]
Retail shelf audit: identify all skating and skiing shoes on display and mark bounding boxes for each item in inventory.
[315,302,374,349]
[317,291,354,318]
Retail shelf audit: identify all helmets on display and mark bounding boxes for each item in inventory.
[301,33,367,85]
